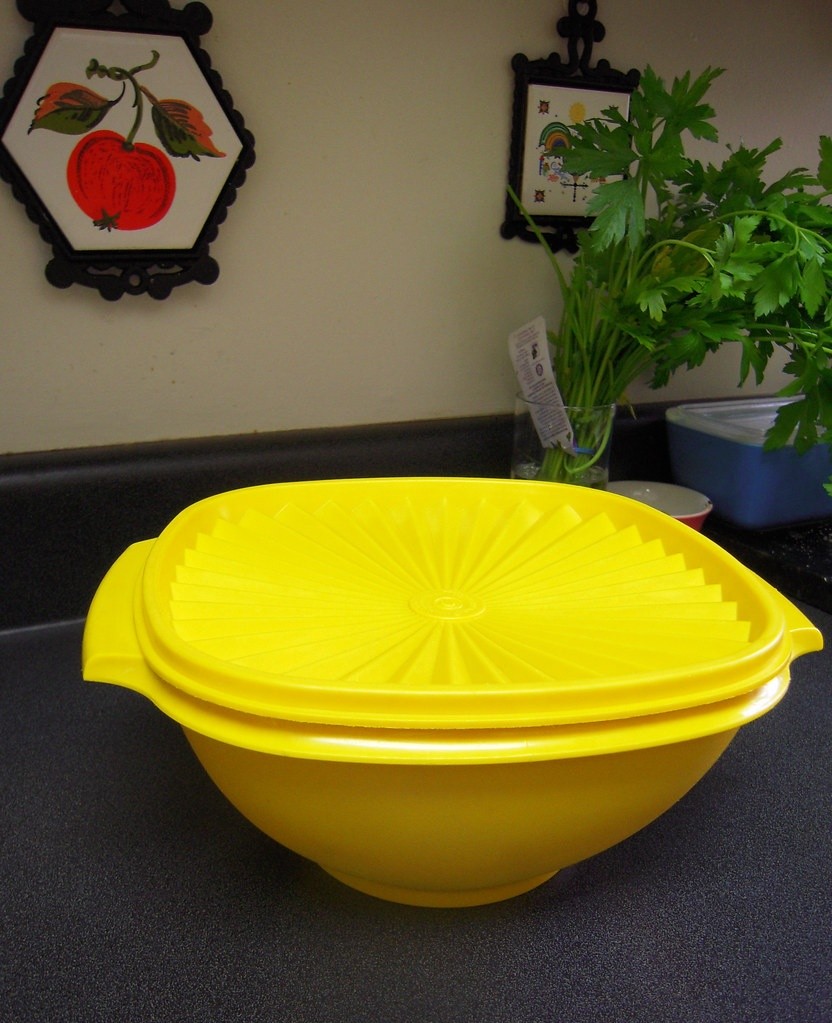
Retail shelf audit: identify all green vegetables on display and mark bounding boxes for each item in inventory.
[509,64,832,500]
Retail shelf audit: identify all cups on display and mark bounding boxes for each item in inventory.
[511,390,617,491]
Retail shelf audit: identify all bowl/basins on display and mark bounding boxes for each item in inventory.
[608,481,713,532]
[82,477,823,907]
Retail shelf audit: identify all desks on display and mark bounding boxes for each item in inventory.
[0,517,832,1023]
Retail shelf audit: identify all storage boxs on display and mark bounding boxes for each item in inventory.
[664,395,832,525]
